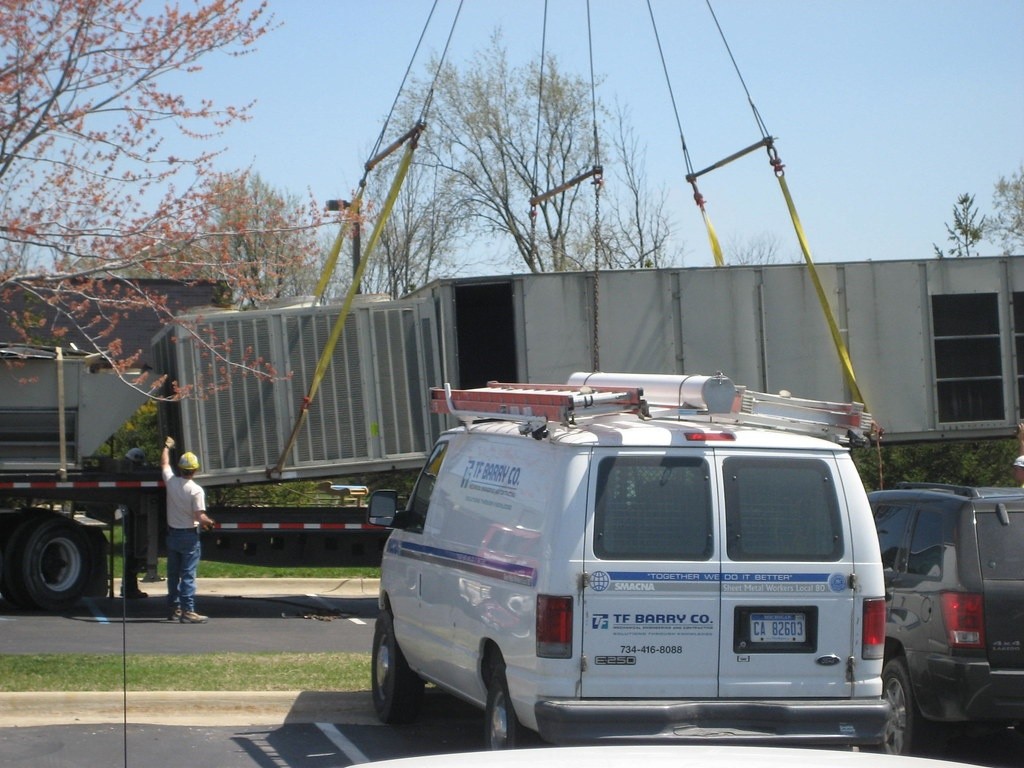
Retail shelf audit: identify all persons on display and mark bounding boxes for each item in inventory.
[161,436,213,624]
[1013,422,1024,488]
[118,447,147,599]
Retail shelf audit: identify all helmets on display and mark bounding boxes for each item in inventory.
[177,452,199,470]
[125,448,145,464]
[1014,456,1024,468]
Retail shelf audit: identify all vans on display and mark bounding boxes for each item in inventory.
[370,369,1024,754]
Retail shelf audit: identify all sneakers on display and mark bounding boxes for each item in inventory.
[180,611,208,623]
[167,606,182,621]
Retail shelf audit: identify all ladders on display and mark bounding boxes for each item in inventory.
[429,380,651,424]
[733,384,870,434]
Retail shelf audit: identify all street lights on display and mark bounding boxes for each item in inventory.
[326,199,364,297]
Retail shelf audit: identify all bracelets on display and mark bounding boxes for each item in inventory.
[163,445,169,448]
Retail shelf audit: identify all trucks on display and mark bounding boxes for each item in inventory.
[0,469,404,617]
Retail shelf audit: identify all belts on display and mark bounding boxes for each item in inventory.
[169,527,196,532]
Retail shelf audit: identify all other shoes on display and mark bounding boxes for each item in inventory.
[120,586,147,598]
